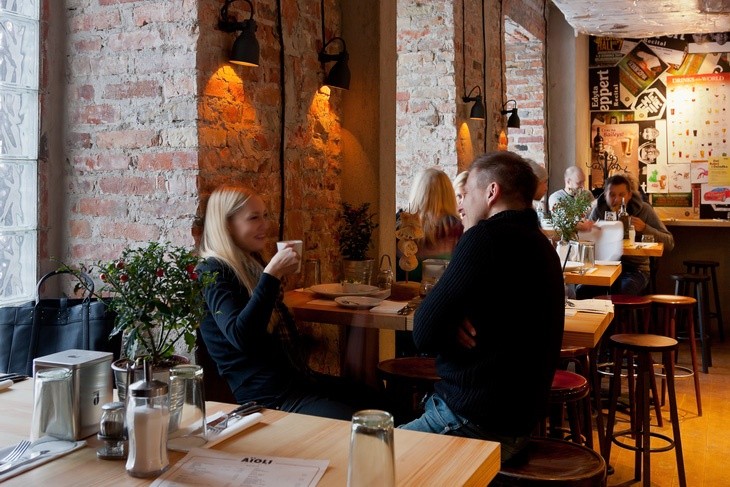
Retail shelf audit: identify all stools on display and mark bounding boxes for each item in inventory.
[543,368,590,445]
[492,433,607,487]
[593,259,719,486]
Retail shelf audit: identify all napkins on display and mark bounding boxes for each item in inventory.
[369,300,408,314]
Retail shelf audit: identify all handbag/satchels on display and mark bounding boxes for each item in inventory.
[0,266,124,374]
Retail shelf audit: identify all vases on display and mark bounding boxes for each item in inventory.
[114,353,190,437]
[341,259,372,283]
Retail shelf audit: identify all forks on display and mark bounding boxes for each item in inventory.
[0,439,30,468]
[206,404,264,437]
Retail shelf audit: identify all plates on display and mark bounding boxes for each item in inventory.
[334,296,383,311]
[310,283,377,298]
[560,261,585,271]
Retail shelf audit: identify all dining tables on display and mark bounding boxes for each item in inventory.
[272,284,616,395]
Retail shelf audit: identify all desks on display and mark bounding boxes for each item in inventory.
[613,240,665,258]
[557,257,622,288]
[0,370,503,487]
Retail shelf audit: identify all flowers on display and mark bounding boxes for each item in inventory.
[52,235,221,367]
[337,201,380,260]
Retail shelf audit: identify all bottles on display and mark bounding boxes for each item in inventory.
[594,127,605,160]
[126,359,171,478]
[618,196,631,248]
[97,402,129,461]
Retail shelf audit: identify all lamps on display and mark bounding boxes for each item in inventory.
[501,100,521,128]
[318,36,351,91]
[464,86,485,120]
[218,0,262,69]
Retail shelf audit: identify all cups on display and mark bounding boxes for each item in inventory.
[557,241,583,274]
[276,240,303,272]
[30,367,80,455]
[166,363,210,452]
[641,233,655,248]
[377,266,393,290]
[620,137,632,164]
[347,409,396,487]
[578,240,596,273]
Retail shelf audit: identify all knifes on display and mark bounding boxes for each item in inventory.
[0,449,50,474]
[185,400,255,440]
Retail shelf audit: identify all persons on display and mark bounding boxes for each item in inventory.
[547,166,597,227]
[448,170,471,221]
[403,167,464,256]
[575,175,676,300]
[523,158,563,251]
[396,151,567,463]
[191,184,396,422]
